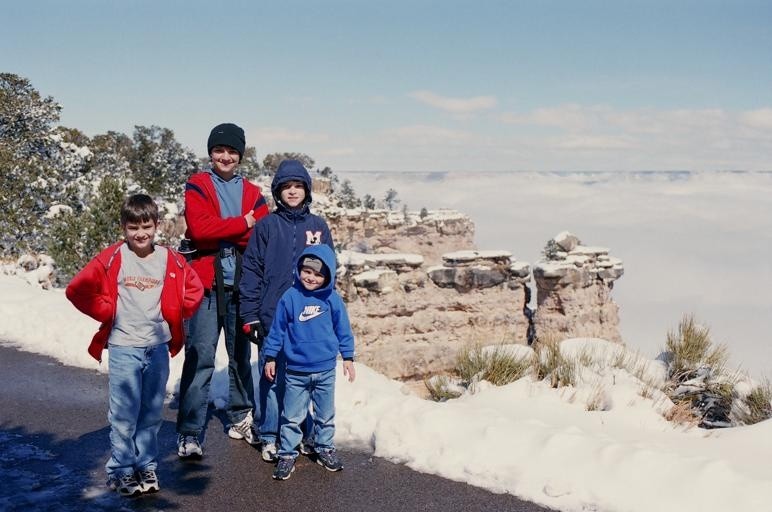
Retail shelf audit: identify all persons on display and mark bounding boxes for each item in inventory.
[66,194,205,499]
[239,159,336,461]
[176,124,265,460]
[264,244,356,480]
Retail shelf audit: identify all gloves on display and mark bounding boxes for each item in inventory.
[243,321,265,343]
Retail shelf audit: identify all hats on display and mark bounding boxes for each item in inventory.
[208,123,245,160]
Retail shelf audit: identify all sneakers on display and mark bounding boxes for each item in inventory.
[177,433,203,459]
[262,443,279,461]
[107,475,143,497]
[317,450,344,471]
[228,408,261,444]
[300,437,315,454]
[136,470,160,493]
[273,458,295,479]
